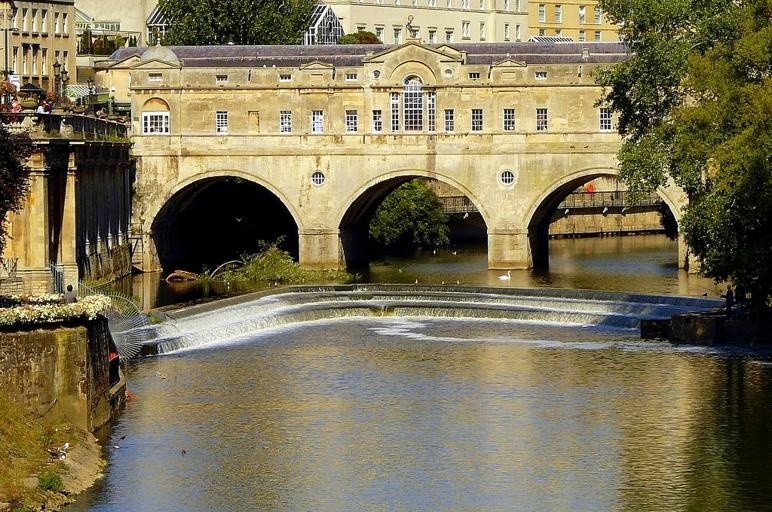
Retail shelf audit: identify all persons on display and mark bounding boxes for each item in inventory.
[725,284,736,312]
[64,285,77,306]
[6,97,127,140]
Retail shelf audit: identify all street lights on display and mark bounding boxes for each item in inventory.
[87,75,96,111]
[1,28,20,103]
[111,96,117,115]
[61,68,68,96]
[51,60,64,115]
